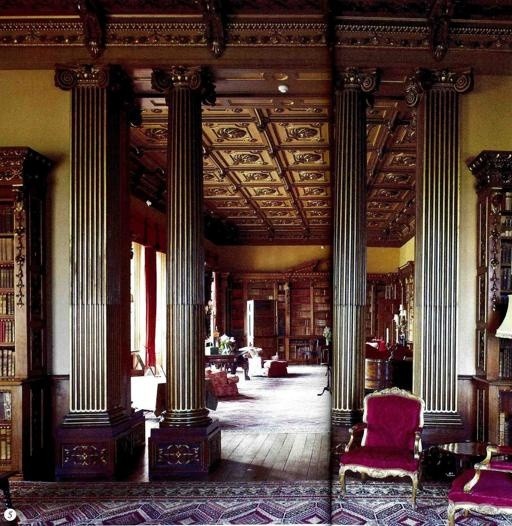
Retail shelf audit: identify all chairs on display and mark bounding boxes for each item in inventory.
[336,387,427,509]
[448,444,512,526]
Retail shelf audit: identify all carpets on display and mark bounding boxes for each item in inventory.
[0,477,512,525]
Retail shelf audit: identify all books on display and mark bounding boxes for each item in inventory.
[498,187,510,466]
[0,201,14,470]
[228,280,387,365]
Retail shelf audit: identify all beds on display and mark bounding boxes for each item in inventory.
[439,441,498,468]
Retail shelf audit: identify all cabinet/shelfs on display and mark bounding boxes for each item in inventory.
[0,145,58,478]
[275,273,332,362]
[394,260,414,345]
[229,273,275,358]
[468,149,512,448]
[366,273,393,341]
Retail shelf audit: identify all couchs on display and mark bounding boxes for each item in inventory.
[263,359,288,377]
[205,369,240,396]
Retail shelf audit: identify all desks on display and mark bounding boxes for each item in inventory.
[205,353,251,380]
